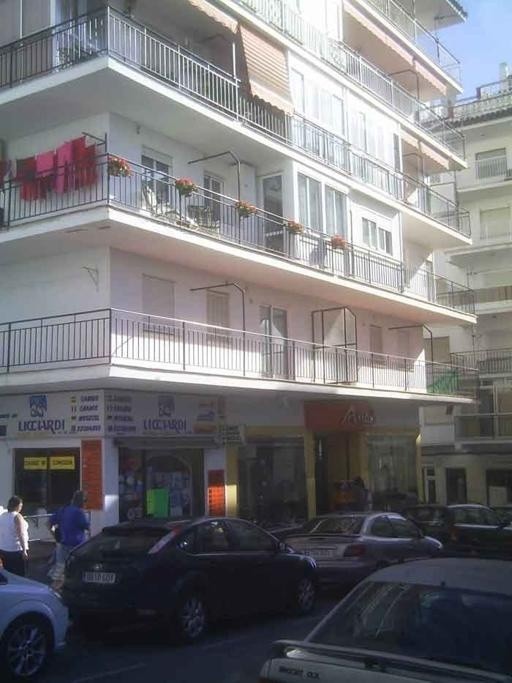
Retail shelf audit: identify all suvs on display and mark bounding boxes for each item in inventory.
[113,444,197,521]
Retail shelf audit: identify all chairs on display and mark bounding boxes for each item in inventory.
[187,205,220,235]
[141,182,198,231]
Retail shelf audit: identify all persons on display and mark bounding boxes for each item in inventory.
[0,495,30,578]
[45,489,92,590]
